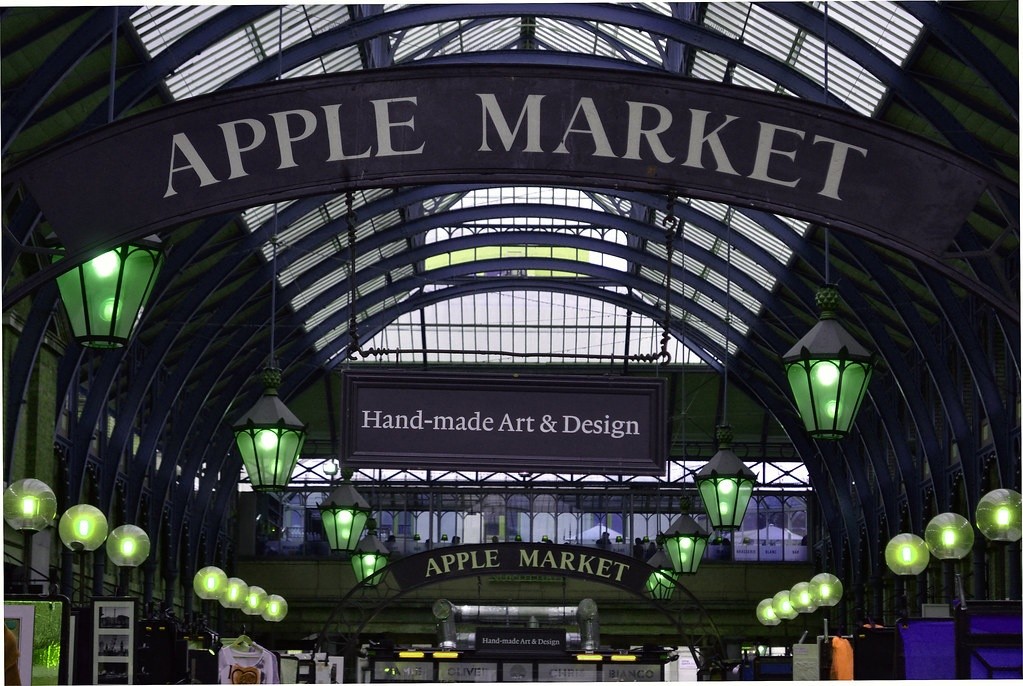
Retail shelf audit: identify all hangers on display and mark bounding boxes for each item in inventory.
[231,635,253,648]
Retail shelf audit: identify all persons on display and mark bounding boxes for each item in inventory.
[452,536,460,543]
[385,536,396,542]
[633,538,656,560]
[596,532,611,544]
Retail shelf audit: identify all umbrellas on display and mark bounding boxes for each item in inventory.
[567,523,629,539]
[748,524,803,541]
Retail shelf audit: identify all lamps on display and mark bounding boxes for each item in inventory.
[106,524,150,596]
[232,5,308,492]
[885,489,1022,617]
[645,532,679,600]
[754,572,844,656]
[690,199,762,531]
[781,227,875,439]
[45,6,172,349]
[59,504,108,607]
[658,193,712,575]
[3,479,58,594]
[352,468,391,587]
[316,188,372,551]
[192,565,288,652]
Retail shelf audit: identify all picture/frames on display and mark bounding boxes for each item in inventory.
[89,596,138,685]
